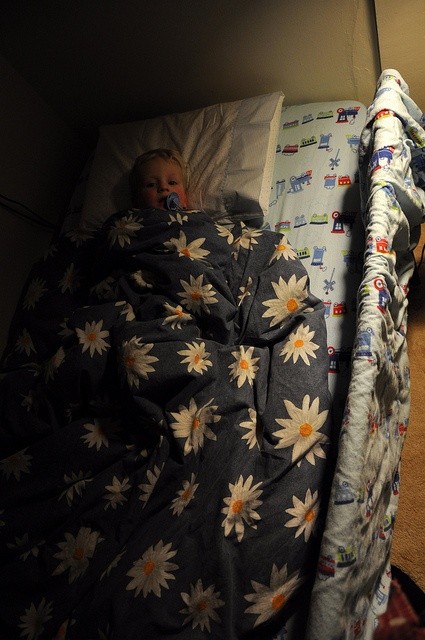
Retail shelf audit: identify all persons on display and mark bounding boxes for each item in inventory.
[129,148,189,210]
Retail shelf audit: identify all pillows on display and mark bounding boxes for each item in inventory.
[79,89,284,228]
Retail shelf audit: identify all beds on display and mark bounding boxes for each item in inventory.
[56,67,405,640]
